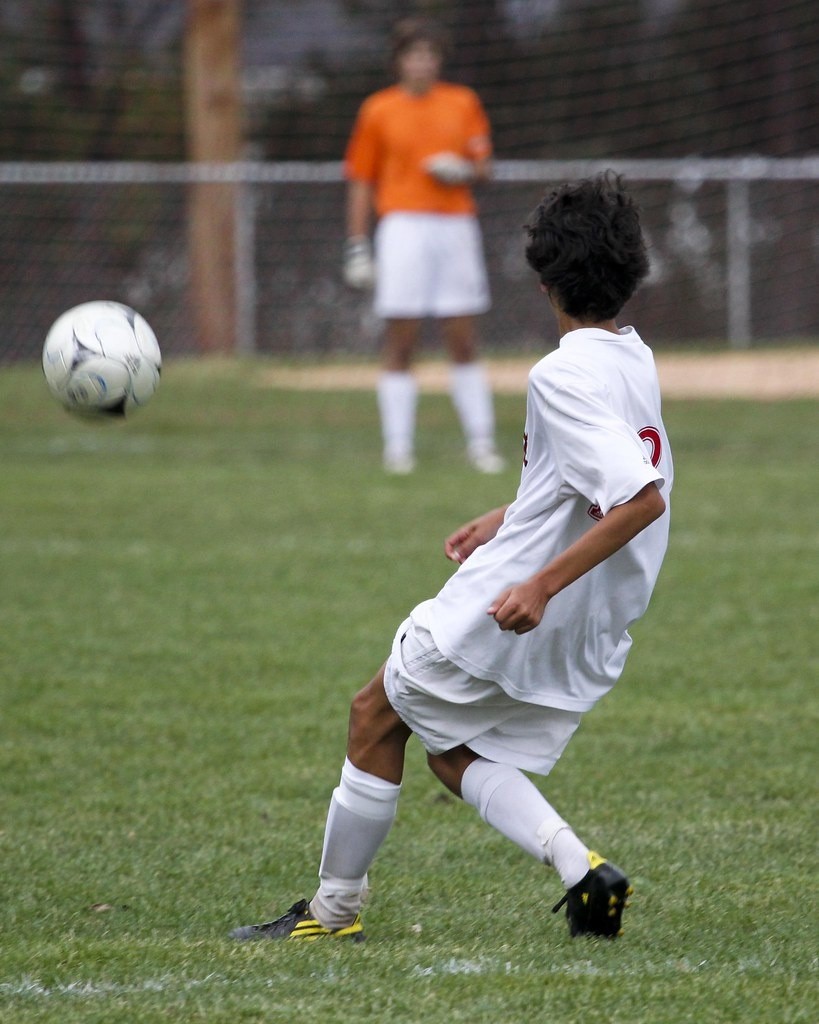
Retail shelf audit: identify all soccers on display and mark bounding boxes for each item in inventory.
[41,299,163,426]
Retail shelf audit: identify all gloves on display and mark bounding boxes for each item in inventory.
[345,244,375,292]
[427,153,474,186]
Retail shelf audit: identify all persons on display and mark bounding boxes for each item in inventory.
[341,15,508,476]
[225,169,674,944]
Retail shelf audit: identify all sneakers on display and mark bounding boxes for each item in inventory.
[228,898,364,944]
[552,849,634,940]
